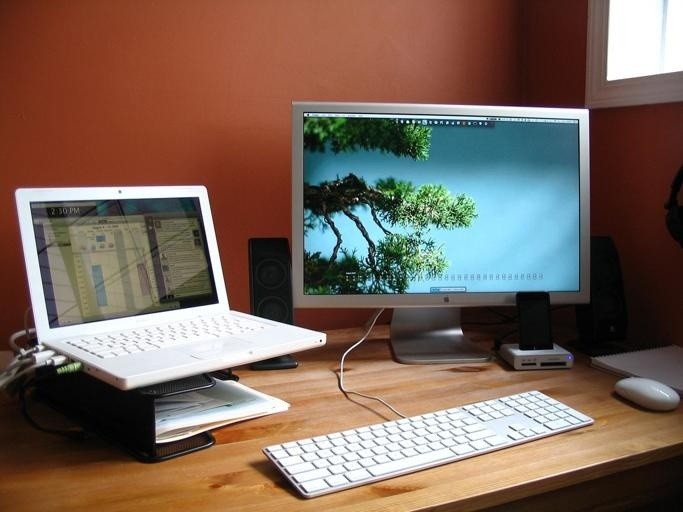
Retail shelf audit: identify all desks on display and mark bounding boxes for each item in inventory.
[0,324,683,512]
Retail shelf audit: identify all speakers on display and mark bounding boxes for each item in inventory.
[248,237,292,369]
[579,235,629,354]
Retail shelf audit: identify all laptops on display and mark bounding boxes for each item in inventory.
[14,185,326,391]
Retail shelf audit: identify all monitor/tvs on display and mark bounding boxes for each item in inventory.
[289,99,593,363]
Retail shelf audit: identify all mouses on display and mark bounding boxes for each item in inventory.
[615,377,680,412]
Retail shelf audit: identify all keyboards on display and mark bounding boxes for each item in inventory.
[262,389,593,498]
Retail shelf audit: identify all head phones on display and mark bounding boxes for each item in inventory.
[664,164,683,244]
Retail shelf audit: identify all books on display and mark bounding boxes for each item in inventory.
[589,344,683,399]
